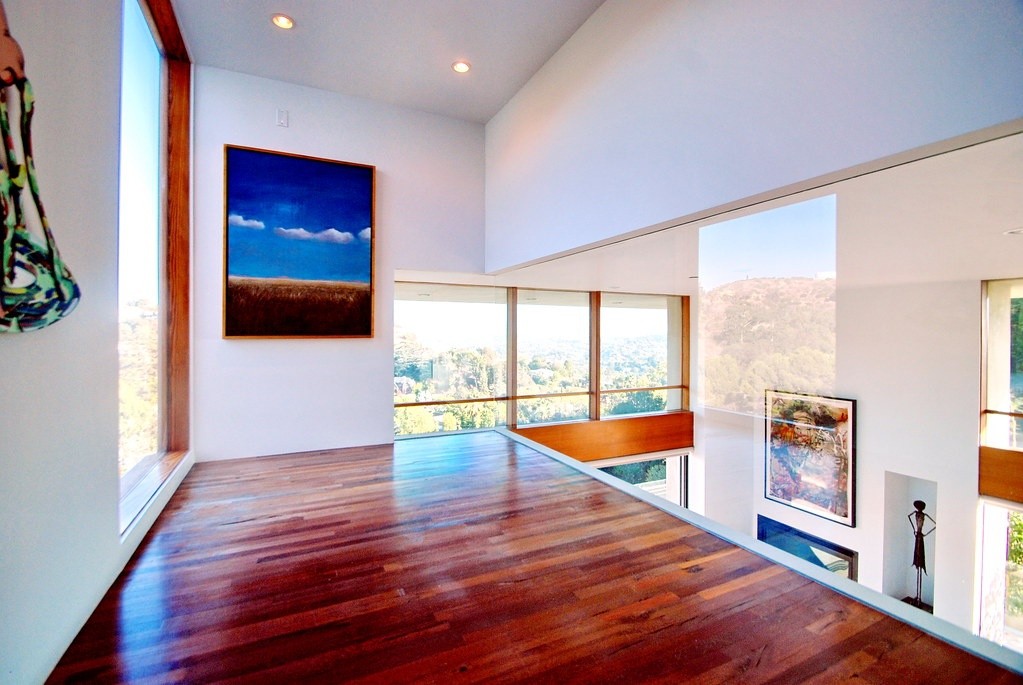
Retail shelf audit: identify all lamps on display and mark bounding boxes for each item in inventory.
[269,12,295,29]
[451,60,471,73]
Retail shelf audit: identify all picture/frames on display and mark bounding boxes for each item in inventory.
[764,388,856,529]
[756,513,858,583]
[223,141,376,342]
